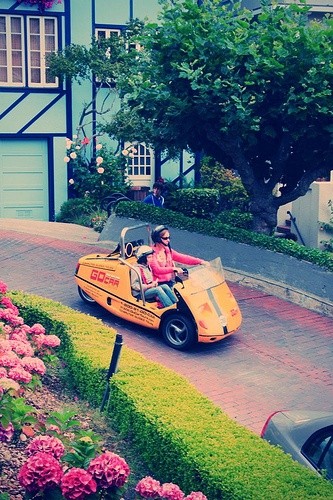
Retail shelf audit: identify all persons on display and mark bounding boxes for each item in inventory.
[131,245,179,307]
[143,183,165,209]
[152,226,211,289]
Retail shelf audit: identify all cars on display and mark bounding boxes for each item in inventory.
[259,407,333,487]
[73,222,242,350]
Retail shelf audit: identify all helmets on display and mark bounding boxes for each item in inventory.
[136,245,153,259]
[151,225,168,243]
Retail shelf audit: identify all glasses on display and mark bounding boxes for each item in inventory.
[161,236,171,240]
